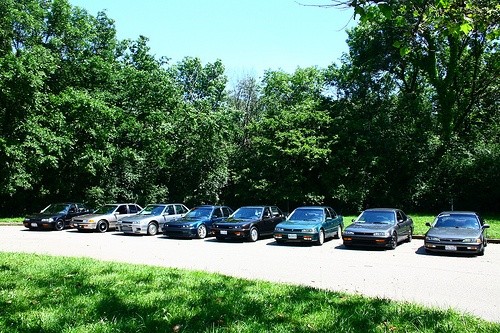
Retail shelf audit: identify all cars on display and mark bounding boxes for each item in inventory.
[210,205,290,243]
[273,206,344,245]
[70,203,153,233]
[114,203,200,236]
[424,210,490,257]
[22,202,95,231]
[341,208,415,250]
[161,204,235,240]
